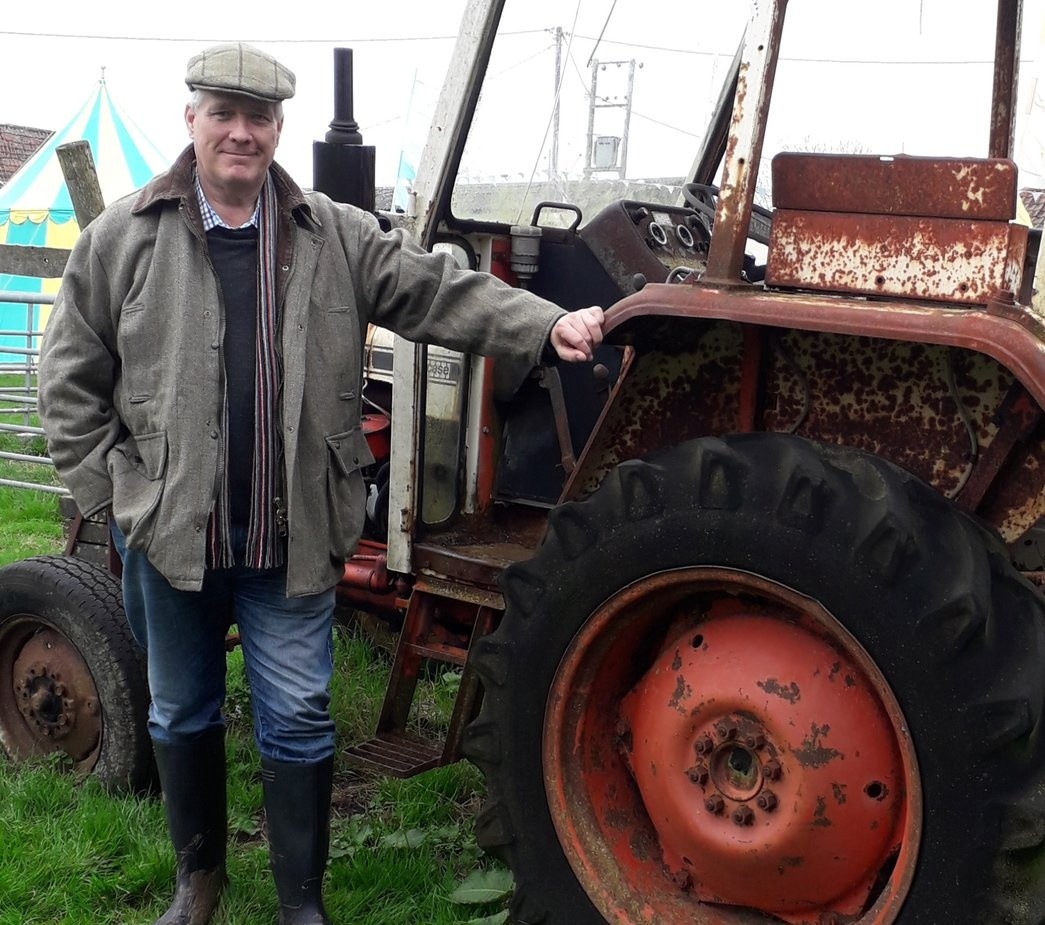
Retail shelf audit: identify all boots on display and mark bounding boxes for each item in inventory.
[260,753,333,925]
[153,731,227,925]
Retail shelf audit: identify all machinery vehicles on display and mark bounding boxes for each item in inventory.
[1,0,1045,925]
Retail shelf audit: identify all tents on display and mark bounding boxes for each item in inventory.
[0,68,156,375]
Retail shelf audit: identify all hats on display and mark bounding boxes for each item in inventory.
[185,43,295,102]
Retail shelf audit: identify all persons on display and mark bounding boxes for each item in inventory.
[40,45,605,925]
